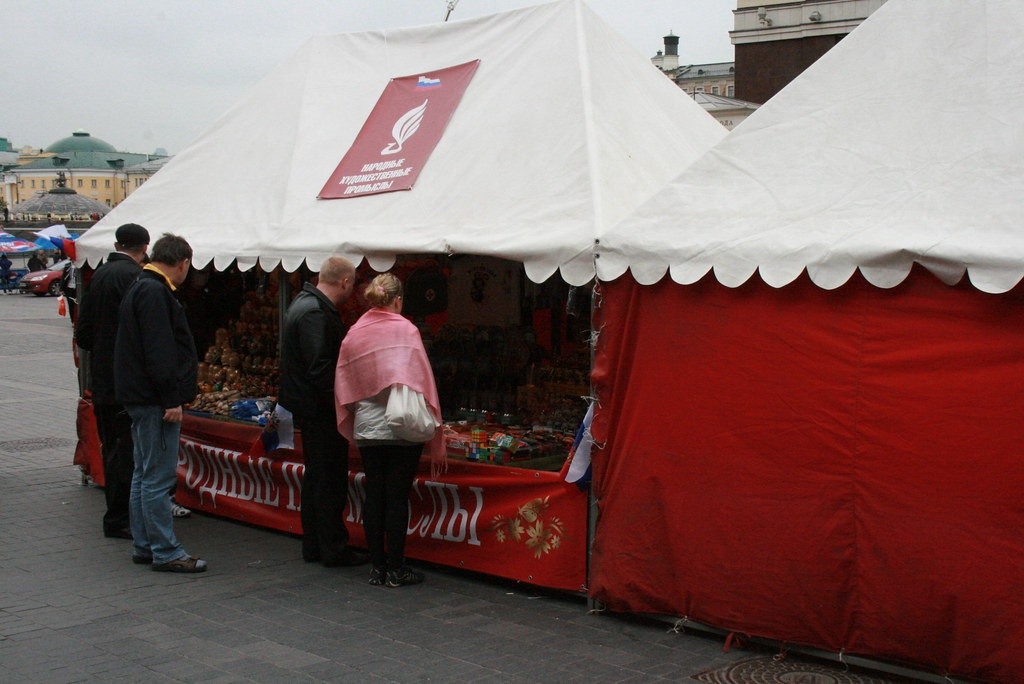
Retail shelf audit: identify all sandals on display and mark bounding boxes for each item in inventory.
[131,552,151,563]
[152,555,207,573]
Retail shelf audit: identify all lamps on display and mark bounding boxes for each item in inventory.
[809,12,820,22]
[757,7,772,26]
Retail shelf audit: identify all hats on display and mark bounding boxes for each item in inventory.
[116,225,150,246]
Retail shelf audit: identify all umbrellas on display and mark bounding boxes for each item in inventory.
[0,231,42,254]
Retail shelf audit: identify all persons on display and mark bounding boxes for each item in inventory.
[76,225,149,541]
[27,253,46,273]
[53,252,60,264]
[61,253,67,261]
[180,264,244,364]
[60,258,76,327]
[111,236,207,573]
[90,212,100,221]
[276,256,369,567]
[0,255,12,295]
[37,250,47,264]
[334,272,446,586]
[46,211,52,223]
[3,205,8,222]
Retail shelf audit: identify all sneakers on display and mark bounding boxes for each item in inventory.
[369,566,387,586]
[386,564,424,588]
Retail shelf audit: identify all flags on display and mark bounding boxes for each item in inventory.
[35,225,76,260]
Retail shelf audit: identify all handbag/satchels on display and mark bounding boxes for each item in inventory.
[385,380,436,442]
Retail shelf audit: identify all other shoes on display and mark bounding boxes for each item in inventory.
[170,501,191,517]
[103,524,133,540]
[302,538,344,568]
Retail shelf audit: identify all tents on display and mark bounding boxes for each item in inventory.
[76,0,731,598]
[595,0,1024,684]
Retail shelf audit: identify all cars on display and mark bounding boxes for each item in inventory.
[17,257,71,297]
